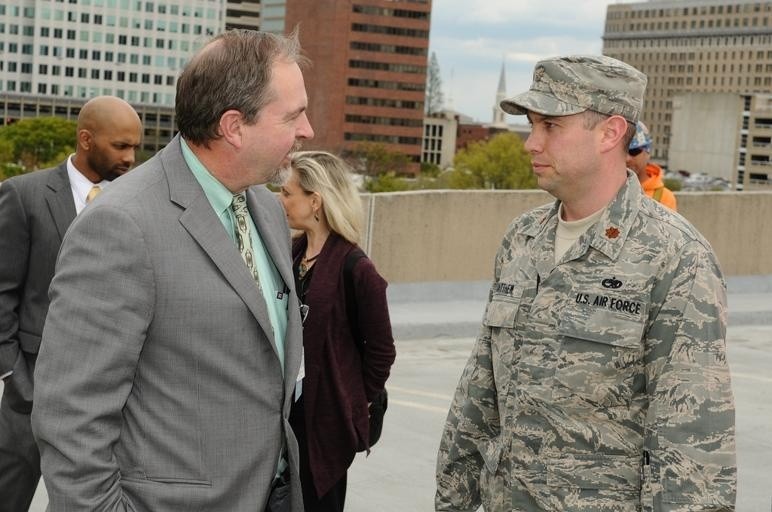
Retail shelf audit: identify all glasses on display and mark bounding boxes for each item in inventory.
[629,147,643,157]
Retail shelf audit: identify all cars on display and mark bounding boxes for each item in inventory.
[664,169,728,190]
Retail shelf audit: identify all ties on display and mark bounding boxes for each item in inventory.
[85,185,105,204]
[230,196,264,302]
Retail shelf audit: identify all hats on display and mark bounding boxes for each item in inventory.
[499,52,651,125]
[628,120,654,150]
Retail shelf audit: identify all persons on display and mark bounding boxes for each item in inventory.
[626,119,677,212]
[269,149,395,512]
[0,97,144,512]
[435,54,737,512]
[29,28,319,512]
